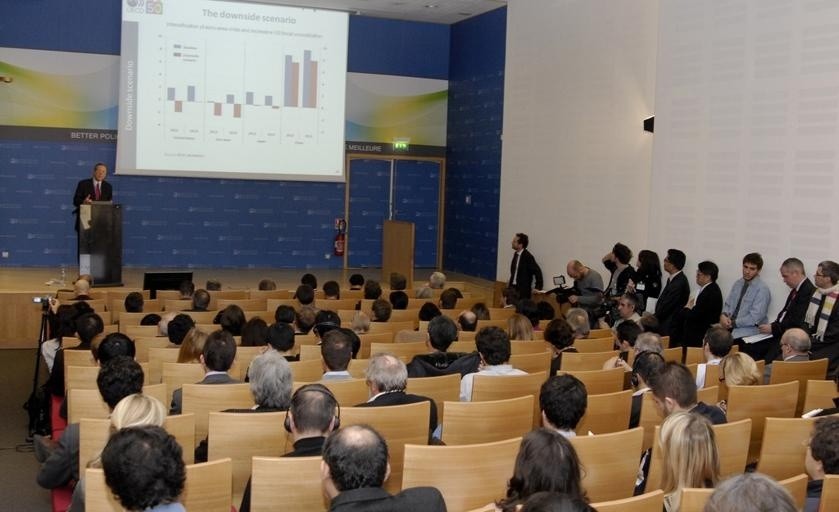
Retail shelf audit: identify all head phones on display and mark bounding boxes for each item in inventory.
[313,322,341,337]
[630,351,665,388]
[284,386,340,434]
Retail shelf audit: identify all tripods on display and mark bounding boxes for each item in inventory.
[23,306,50,432]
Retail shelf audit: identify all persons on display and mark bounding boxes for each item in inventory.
[391,272,407,290]
[614,320,642,349]
[539,374,589,436]
[58,331,113,420]
[195,349,294,464]
[66,279,95,300]
[515,298,541,331]
[714,351,760,414]
[438,289,456,309]
[389,290,409,309]
[566,260,603,330]
[634,361,728,495]
[355,280,382,310]
[238,382,342,512]
[296,285,320,311]
[798,260,839,372]
[124,292,144,313]
[259,321,300,361]
[319,330,354,381]
[349,274,365,290]
[742,258,816,365]
[213,310,224,324]
[352,311,371,334]
[448,287,464,298]
[602,242,635,297]
[159,312,178,336]
[320,423,448,511]
[179,280,194,300]
[40,305,79,374]
[703,471,802,511]
[603,330,664,371]
[73,162,113,265]
[696,326,733,389]
[72,273,92,290]
[764,327,811,384]
[657,412,720,511]
[140,314,161,325]
[471,302,491,320]
[460,325,528,401]
[295,307,316,335]
[27,313,105,441]
[515,492,596,511]
[221,304,247,336]
[416,288,433,299]
[802,419,839,512]
[802,365,839,417]
[206,279,222,291]
[352,352,438,446]
[97,332,137,367]
[429,271,446,289]
[65,392,166,512]
[406,314,480,379]
[628,351,666,428]
[167,314,196,348]
[36,352,148,488]
[506,313,534,340]
[598,293,644,331]
[655,249,690,319]
[48,295,95,314]
[413,303,441,331]
[101,424,186,511]
[369,299,392,322]
[181,289,210,312]
[669,261,723,347]
[241,315,268,346]
[259,279,276,290]
[457,310,477,331]
[719,253,771,343]
[507,232,544,299]
[274,304,296,324]
[537,300,555,319]
[322,280,340,300]
[543,318,577,376]
[167,330,245,414]
[495,426,588,512]
[177,328,209,363]
[313,309,341,345]
[626,249,662,315]
[500,286,521,309]
[565,307,590,339]
[300,273,317,290]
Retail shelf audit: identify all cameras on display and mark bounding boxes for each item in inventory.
[32,295,52,306]
[553,275,582,304]
[600,300,618,315]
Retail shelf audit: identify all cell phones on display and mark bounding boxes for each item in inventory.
[755,322,764,327]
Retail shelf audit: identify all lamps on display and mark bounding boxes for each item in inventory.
[643,115,654,134]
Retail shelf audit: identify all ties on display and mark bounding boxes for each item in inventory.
[514,254,518,270]
[95,184,100,201]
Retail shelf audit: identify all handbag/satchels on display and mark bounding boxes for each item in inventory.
[28,390,50,435]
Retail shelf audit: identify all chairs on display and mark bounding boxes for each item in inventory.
[440,396,536,447]
[465,292,745,367]
[470,371,549,429]
[51,383,170,448]
[704,360,766,402]
[556,366,626,397]
[643,418,753,495]
[401,437,522,512]
[405,373,461,426]
[179,383,258,448]
[683,363,699,383]
[803,380,839,418]
[49,412,196,512]
[725,381,801,468]
[769,359,829,417]
[513,489,666,512]
[753,413,839,483]
[250,455,326,512]
[336,401,431,497]
[574,389,633,437]
[818,473,839,511]
[207,410,301,512]
[562,427,644,504]
[85,459,232,512]
[678,474,808,512]
[291,378,370,409]
[638,384,720,453]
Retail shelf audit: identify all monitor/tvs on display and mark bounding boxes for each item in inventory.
[143,272,193,299]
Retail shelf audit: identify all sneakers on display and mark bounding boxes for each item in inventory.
[33,435,60,463]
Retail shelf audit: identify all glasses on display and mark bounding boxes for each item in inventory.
[816,271,824,276]
[718,377,725,382]
